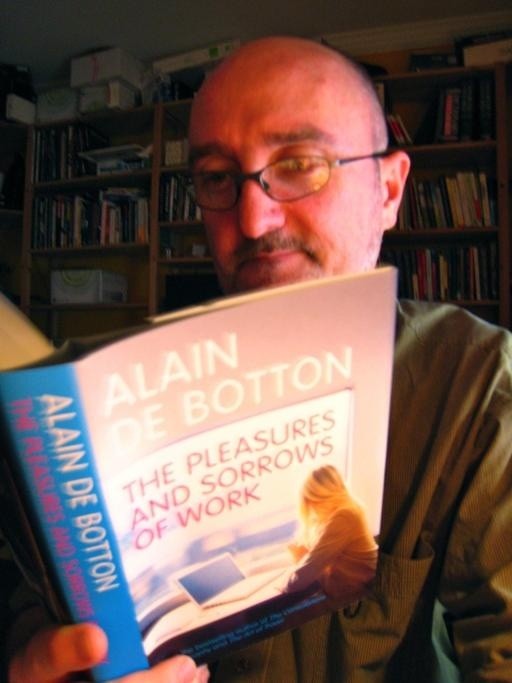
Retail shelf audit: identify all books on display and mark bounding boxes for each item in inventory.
[371,79,497,147]
[1,264,402,683]
[160,173,211,258]
[388,167,499,230]
[380,241,500,301]
[165,136,189,165]
[33,189,150,249]
[33,121,152,183]
[25,78,203,125]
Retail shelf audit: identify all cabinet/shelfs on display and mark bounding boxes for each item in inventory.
[1,114,28,316]
[18,100,154,347]
[376,59,511,337]
[148,90,219,316]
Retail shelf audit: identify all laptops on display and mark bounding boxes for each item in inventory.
[177,553,284,611]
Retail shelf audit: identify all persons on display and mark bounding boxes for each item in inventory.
[270,454,385,604]
[1,25,511,682]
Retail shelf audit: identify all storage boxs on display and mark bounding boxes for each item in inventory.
[451,27,512,67]
[46,267,136,305]
[1,35,242,126]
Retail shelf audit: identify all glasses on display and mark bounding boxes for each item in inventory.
[175,148,391,214]
